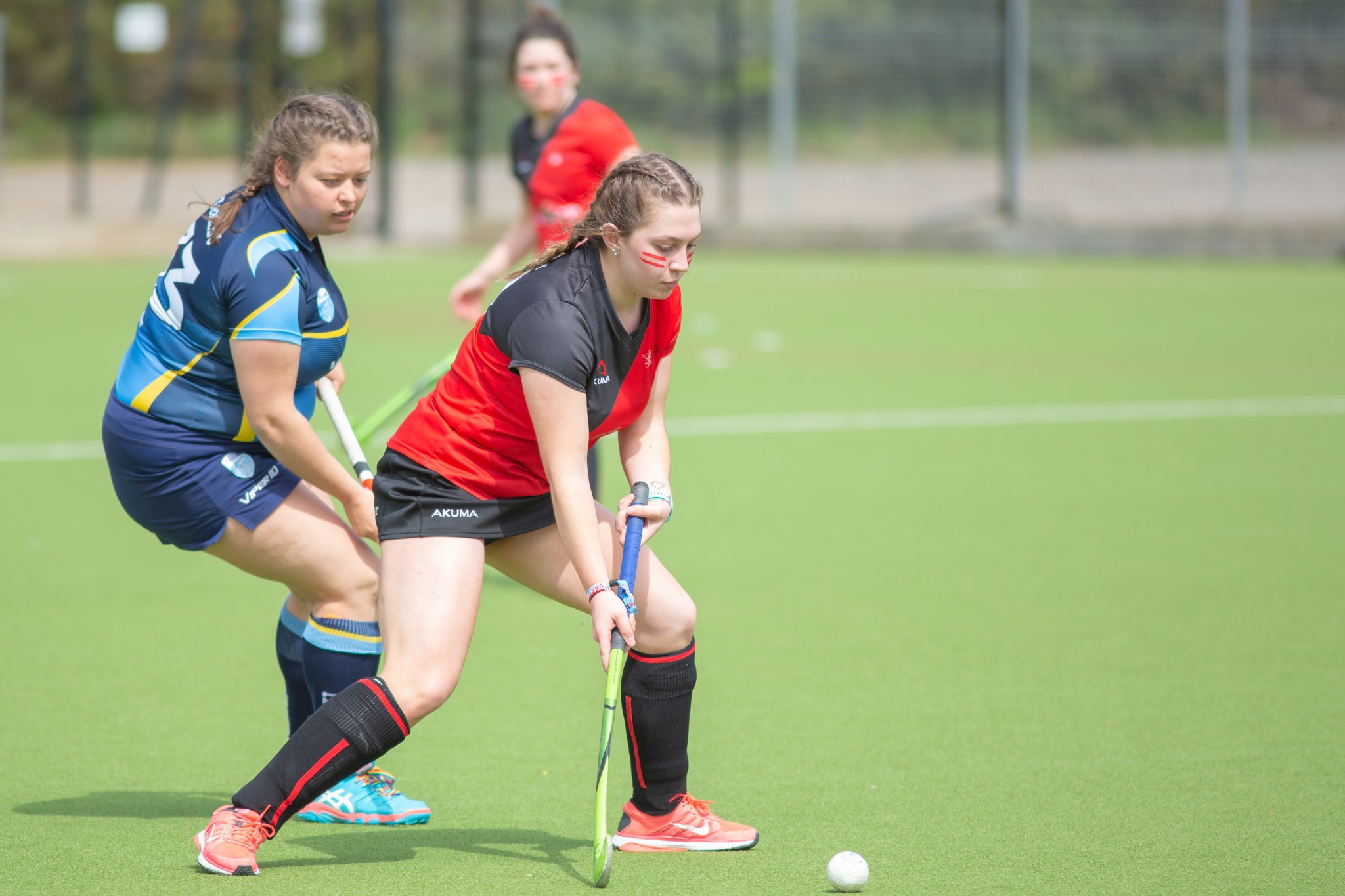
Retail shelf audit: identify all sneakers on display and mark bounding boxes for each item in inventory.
[194,803,277,877]
[296,761,431,826]
[611,790,758,852]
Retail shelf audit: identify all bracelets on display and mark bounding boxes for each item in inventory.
[648,493,673,521]
[587,582,614,603]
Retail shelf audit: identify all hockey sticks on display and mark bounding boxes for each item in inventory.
[354,344,458,444]
[317,376,375,491]
[591,481,650,888]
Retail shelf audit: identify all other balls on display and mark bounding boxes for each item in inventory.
[828,851,869,893]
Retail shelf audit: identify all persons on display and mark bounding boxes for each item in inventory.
[189,152,757,875]
[101,93,432,826]
[452,9,643,318]
